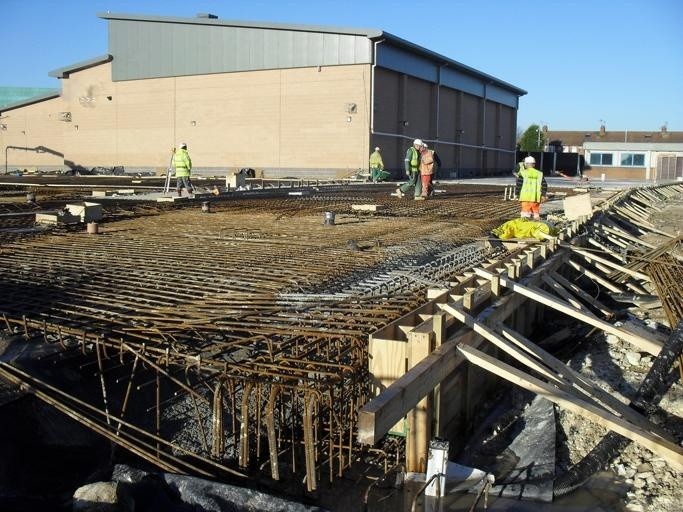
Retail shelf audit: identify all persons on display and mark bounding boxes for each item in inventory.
[416,143,442,199]
[511,161,525,178]
[516,156,548,218]
[172,144,192,192]
[369,146,384,184]
[395,139,423,199]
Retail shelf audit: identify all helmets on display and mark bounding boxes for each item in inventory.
[414,138,428,148]
[180,143,187,149]
[525,156,537,164]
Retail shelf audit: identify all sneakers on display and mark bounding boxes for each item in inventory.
[395,188,402,198]
[415,196,425,201]
